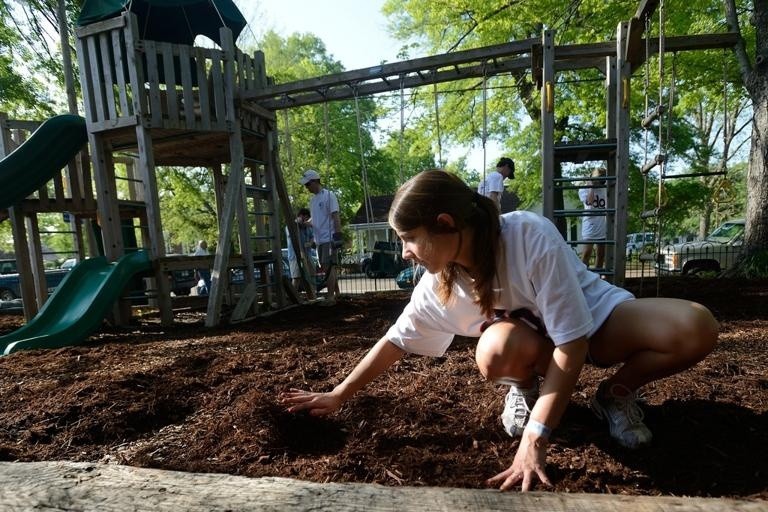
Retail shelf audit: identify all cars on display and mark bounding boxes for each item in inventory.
[342,250,373,268]
[391,262,420,290]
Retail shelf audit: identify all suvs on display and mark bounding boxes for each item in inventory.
[652,215,748,279]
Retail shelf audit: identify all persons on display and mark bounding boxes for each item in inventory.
[578,167,606,268]
[281,172,719,493]
[285,208,315,288]
[195,240,211,292]
[478,158,515,214]
[299,170,344,306]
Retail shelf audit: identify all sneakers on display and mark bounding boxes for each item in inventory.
[594,386,650,449]
[502,383,543,440]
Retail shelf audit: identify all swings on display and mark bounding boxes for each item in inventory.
[285,90,338,286]
[352,81,408,278]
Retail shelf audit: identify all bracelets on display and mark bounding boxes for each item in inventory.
[528,420,552,441]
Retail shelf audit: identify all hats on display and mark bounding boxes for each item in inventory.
[298,168,320,186]
[498,157,515,179]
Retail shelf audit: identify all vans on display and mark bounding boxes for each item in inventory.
[624,231,661,261]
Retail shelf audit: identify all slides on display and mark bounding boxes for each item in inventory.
[0,114,86,208]
[0,251,149,355]
